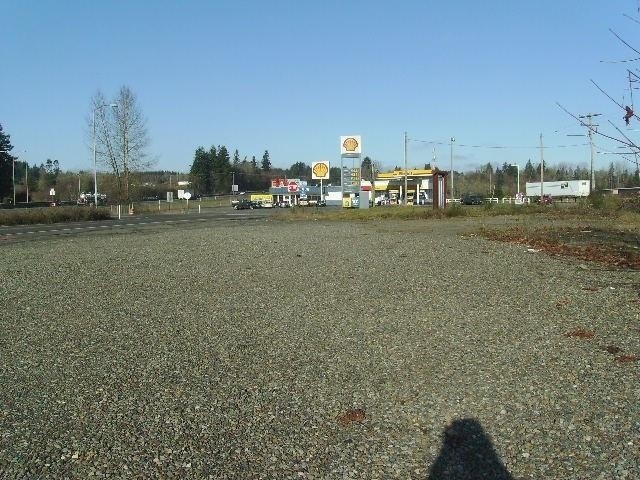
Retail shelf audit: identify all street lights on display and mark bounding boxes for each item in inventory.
[450,137,455,199]
[93,104,118,209]
[12,149,27,206]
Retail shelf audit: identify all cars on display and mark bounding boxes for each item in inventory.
[232,198,326,210]
[459,197,482,205]
